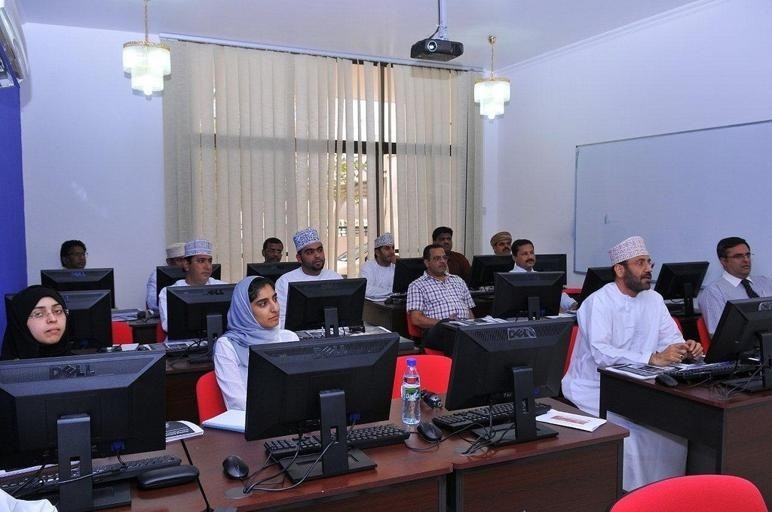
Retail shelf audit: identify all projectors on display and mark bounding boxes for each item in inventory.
[411,38,466,64]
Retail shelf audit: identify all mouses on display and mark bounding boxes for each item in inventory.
[222,455,250,479]
[137,311,146,318]
[416,422,442,443]
[384,298,393,304]
[136,344,154,351]
[656,373,680,387]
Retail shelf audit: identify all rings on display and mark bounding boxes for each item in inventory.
[679,354,682,360]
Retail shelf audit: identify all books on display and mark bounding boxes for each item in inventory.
[536,408,607,434]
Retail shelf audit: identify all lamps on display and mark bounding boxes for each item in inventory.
[119,1,173,99]
[471,34,512,121]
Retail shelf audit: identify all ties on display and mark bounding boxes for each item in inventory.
[741,279,759,298]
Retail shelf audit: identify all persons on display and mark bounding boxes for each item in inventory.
[1,286,80,361]
[146,242,197,316]
[55,238,90,267]
[562,236,705,492]
[510,239,580,313]
[486,230,513,258]
[360,232,405,301]
[426,227,474,276]
[269,228,344,329]
[696,237,772,341]
[404,243,475,329]
[259,238,290,262]
[209,275,302,416]
[155,238,224,333]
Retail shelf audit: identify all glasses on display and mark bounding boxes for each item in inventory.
[30,307,69,320]
[66,250,86,257]
[627,262,655,269]
[269,249,282,253]
[726,253,754,258]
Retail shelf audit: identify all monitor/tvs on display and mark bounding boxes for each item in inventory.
[0,350,167,511]
[470,255,515,287]
[531,254,567,285]
[443,318,575,448]
[247,261,301,283]
[243,333,401,484]
[576,266,615,309]
[493,271,565,320]
[392,257,427,292]
[283,277,367,336]
[165,283,239,363]
[156,263,221,306]
[40,268,115,309]
[704,295,772,392]
[4,290,114,347]
[654,261,709,317]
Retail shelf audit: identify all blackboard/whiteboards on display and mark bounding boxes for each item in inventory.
[574,119,772,290]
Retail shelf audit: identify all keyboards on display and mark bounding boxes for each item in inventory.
[433,401,552,432]
[470,289,492,295]
[264,421,411,461]
[666,303,684,309]
[390,294,406,298]
[168,345,206,354]
[296,330,324,339]
[665,363,752,383]
[0,454,181,502]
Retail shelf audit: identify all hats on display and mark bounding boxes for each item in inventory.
[609,236,649,268]
[375,233,394,248]
[294,228,321,252]
[490,232,512,248]
[167,242,187,258]
[183,240,213,256]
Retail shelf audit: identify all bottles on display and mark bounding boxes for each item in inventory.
[401,358,420,425]
